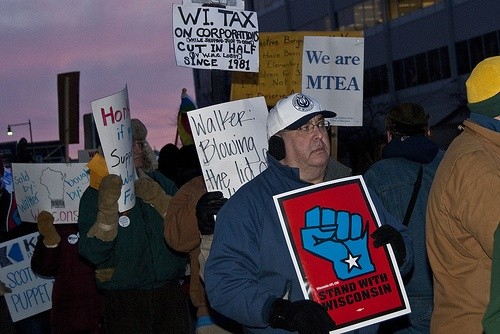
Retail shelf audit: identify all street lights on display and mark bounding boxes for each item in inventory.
[6,119,34,142]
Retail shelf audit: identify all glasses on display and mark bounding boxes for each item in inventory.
[279,120,331,134]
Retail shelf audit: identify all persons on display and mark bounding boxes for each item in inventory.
[426,56,500,334]
[31,93,446,334]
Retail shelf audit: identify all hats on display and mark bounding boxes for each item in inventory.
[385,102,430,134]
[267,93,337,140]
[129,119,147,140]
[466,56,500,117]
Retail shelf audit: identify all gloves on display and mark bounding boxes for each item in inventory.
[371,224,406,267]
[268,298,336,334]
[86,174,122,243]
[196,191,229,235]
[134,177,173,220]
[38,211,61,244]
